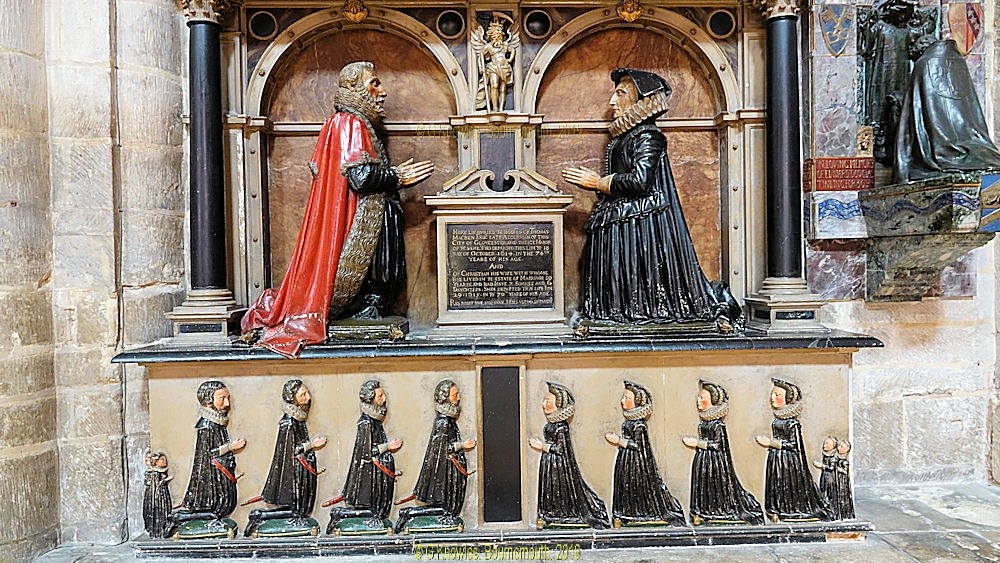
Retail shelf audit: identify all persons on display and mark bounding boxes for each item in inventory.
[143,451,175,539]
[857,0,1000,181]
[529,380,612,529]
[605,381,688,526]
[562,67,745,327]
[244,380,327,537]
[327,380,403,537]
[683,380,765,525]
[394,379,476,534]
[469,15,520,113]
[812,435,856,520]
[240,62,435,357]
[164,381,246,538]
[756,378,825,521]
